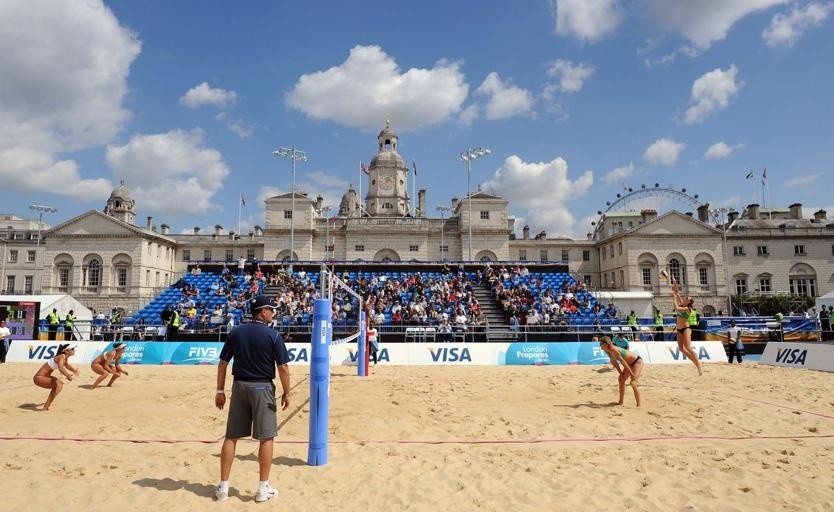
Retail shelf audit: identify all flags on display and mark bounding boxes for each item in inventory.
[759,167,770,189]
[743,167,755,184]
[358,159,370,176]
[238,192,247,209]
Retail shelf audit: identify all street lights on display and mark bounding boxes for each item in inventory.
[270,145,310,260]
[27,205,56,295]
[707,206,739,317]
[314,206,333,262]
[435,205,451,262]
[459,145,493,261]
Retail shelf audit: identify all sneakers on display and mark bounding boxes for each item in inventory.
[216,488,229,501]
[255,488,280,503]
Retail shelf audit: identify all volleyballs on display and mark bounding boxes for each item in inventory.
[658,270,668,281]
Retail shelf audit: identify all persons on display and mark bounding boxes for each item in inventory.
[210,294,293,503]
[62,309,77,340]
[45,308,61,340]
[160,256,620,332]
[607,330,630,373]
[739,305,747,317]
[599,335,646,410]
[774,305,834,332]
[95,309,146,341]
[88,342,129,390]
[725,318,744,365]
[669,271,705,377]
[365,319,379,367]
[0,317,12,364]
[626,304,700,341]
[32,343,80,411]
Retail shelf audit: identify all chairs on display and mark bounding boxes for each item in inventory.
[610,325,654,341]
[404,325,435,342]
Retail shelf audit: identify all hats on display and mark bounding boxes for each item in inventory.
[249,296,279,311]
[61,344,78,353]
[114,343,128,350]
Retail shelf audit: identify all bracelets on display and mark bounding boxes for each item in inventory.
[215,389,224,395]
[282,393,291,397]
